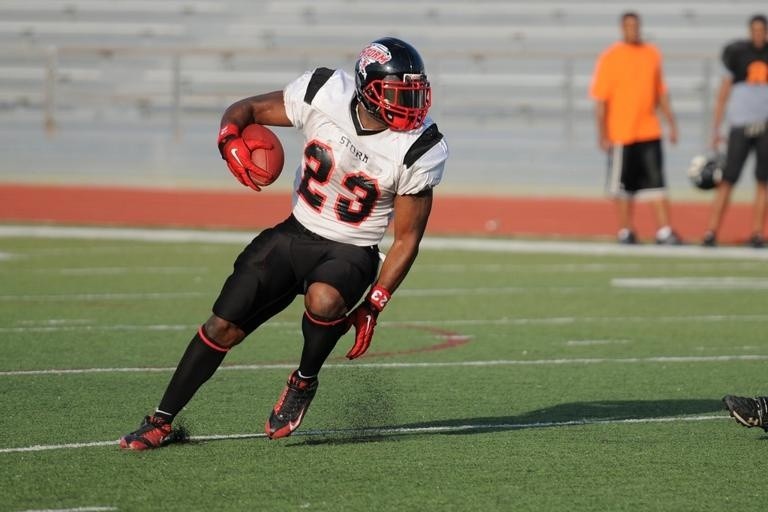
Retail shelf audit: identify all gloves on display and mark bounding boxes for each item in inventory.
[343,286,394,360]
[216,125,275,191]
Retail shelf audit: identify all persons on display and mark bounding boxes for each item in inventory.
[723,388,768,436]
[698,12,768,250]
[587,11,682,247]
[117,35,448,453]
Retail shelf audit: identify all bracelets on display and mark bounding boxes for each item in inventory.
[365,285,393,310]
[217,121,241,141]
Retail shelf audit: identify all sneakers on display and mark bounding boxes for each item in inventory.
[722,392,766,433]
[121,416,175,451]
[615,224,767,251]
[264,367,319,441]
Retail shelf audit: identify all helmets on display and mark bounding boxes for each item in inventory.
[355,35,431,131]
[690,153,725,189]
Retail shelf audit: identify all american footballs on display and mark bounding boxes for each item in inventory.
[241,124,284,186]
